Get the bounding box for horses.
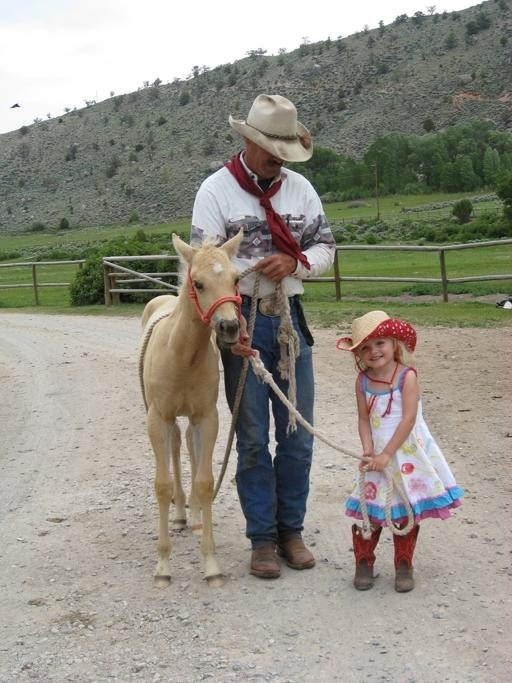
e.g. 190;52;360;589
138;226;246;591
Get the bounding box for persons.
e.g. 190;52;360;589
186;92;339;580
335;308;464;592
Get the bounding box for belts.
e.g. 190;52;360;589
249;295;296;318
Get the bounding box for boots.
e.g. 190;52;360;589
351;524;381;590
394;525;418;592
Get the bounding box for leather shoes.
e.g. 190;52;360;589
250;544;280;578
277;537;314;570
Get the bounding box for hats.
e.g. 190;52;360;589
337;311;418;353
228;94;314;163
